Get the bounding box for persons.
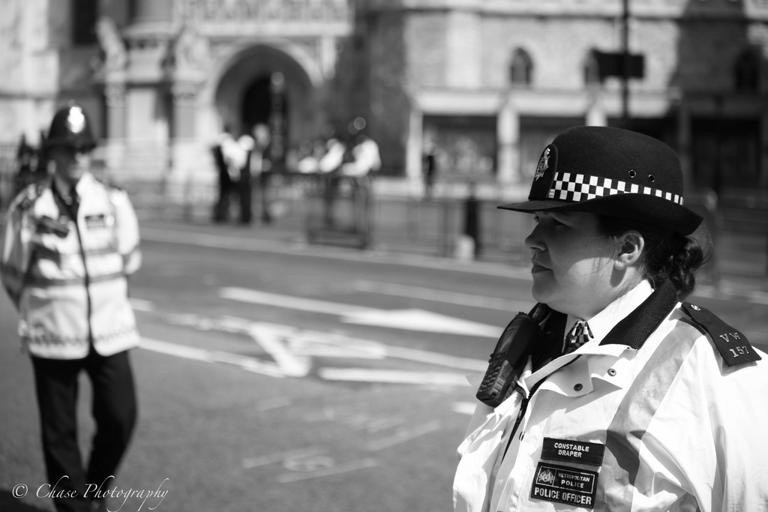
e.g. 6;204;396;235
13;132;51;195
210;121;381;249
462;181;482;261
421;143;440;198
451;126;768;512
0;103;143;512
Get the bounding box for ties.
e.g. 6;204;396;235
564;321;593;354
69;185;79;221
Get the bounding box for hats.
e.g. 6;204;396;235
497;127;704;238
45;106;96;150
